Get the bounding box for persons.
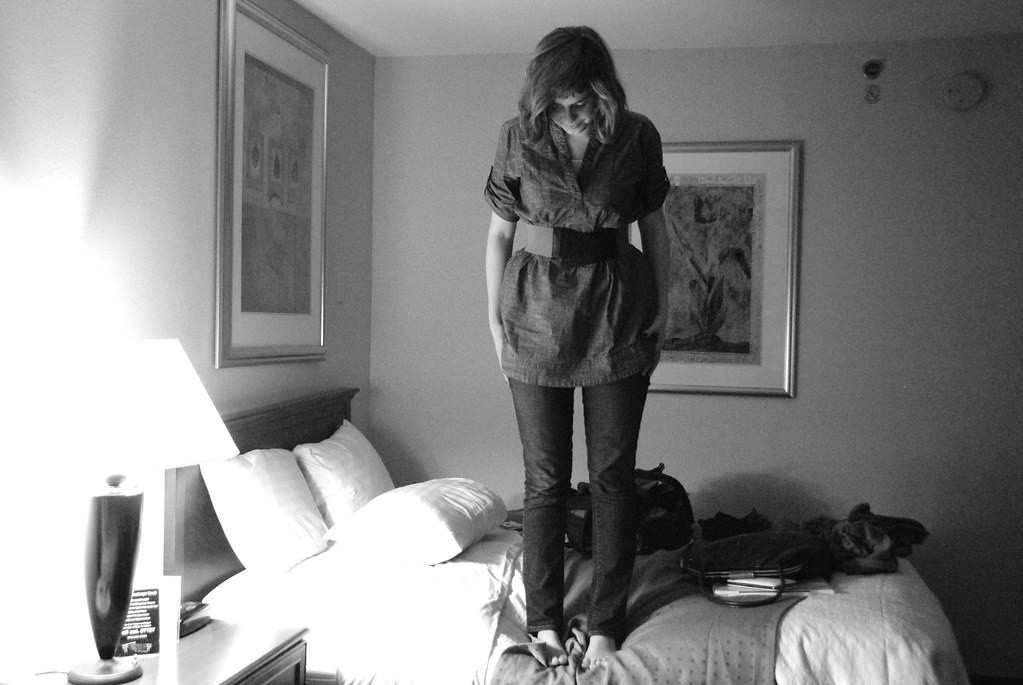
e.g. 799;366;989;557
486;26;671;670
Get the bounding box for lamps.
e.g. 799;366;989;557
57;337;239;685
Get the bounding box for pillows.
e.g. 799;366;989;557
322;477;508;567
292;418;395;530
200;448;330;577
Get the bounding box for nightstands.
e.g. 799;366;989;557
0;617;310;685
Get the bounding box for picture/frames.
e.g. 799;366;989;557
627;139;806;399
213;0;332;369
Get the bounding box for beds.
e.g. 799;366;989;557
163;387;969;685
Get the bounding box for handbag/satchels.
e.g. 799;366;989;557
685;530;832;607
565;465;694;558
